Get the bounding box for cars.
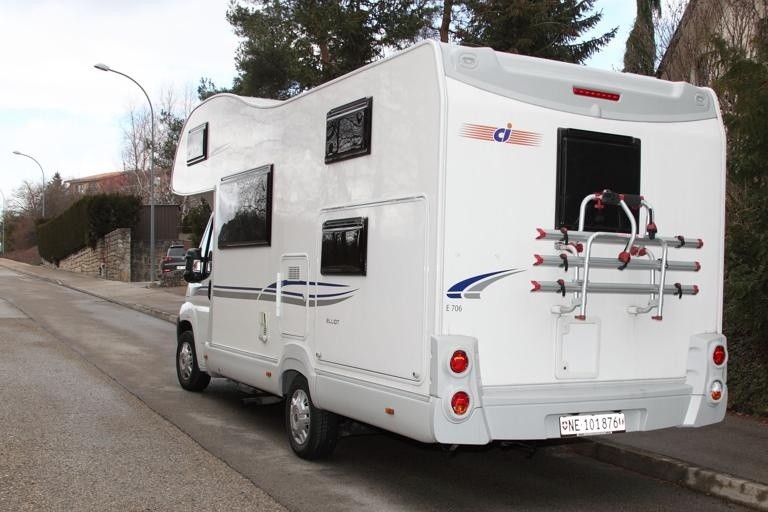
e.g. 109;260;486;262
160;245;187;279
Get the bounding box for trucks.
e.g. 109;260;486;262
170;38;728;461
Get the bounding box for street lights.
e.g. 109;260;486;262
94;62;156;282
13;151;45;216
